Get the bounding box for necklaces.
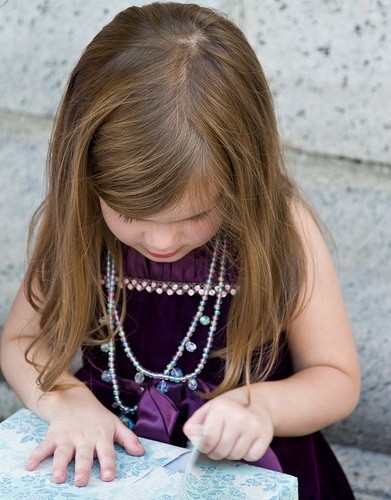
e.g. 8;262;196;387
98;231;229;429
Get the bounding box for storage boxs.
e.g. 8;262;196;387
0;407;298;500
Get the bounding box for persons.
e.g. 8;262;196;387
1;0;363;500
